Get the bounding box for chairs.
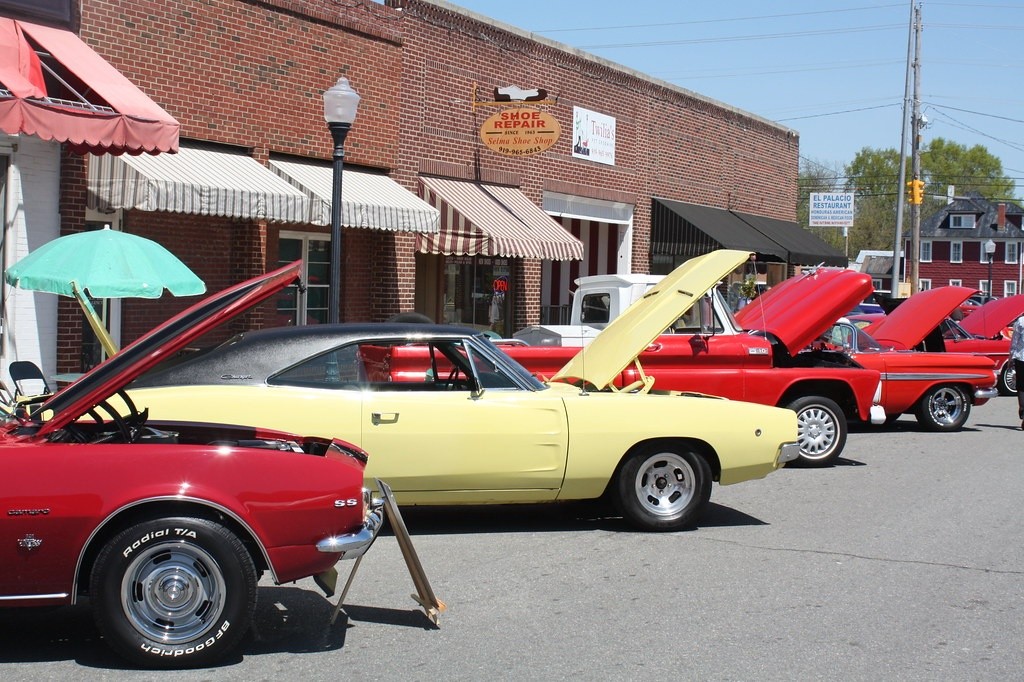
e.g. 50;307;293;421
8;361;55;406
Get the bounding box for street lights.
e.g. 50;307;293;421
320;75;361;325
985;238;996;300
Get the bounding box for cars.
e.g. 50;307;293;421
792;285;999;432
511;272;744;350
358;265;888;470
14;249;803;532
0;257;386;669
842;289;1024;398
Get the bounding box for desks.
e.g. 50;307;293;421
50;373;86;382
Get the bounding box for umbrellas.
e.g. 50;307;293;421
4;224;207;364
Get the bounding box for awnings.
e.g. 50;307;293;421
651;198;848;267
0;9;181;157
414;175;585;262
85;140;440;235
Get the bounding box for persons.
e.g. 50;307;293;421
736;285;772;313
1008;314;1024;430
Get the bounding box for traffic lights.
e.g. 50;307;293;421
906;180;925;205
